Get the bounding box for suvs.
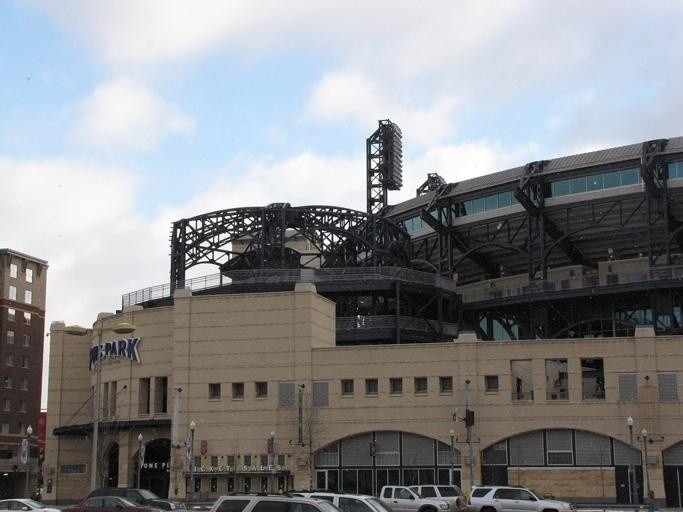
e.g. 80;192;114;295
293;491;393;511
86;487;175;510
465;485;576;512
210;495;341;511
407;484;466;511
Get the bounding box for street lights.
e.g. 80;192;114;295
269;430;276;493
626;415;639;511
23;424;32;497
61;322;135;491
189;420;195;508
641;427;652;492
137;433;143;488
447;430;455;484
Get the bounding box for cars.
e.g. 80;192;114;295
62;495;165;512
0;498;62;512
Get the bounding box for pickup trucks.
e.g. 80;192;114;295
379;484;451;512
